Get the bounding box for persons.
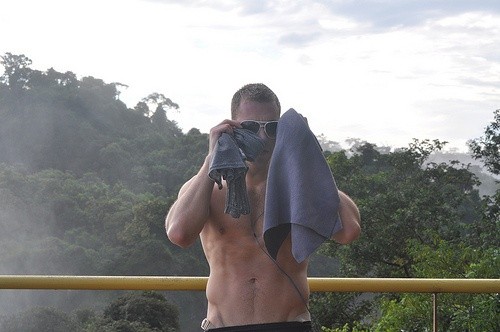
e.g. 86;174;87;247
165;84;361;332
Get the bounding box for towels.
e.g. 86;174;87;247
263;108;344;265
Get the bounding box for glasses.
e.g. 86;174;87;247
240;120;278;138
